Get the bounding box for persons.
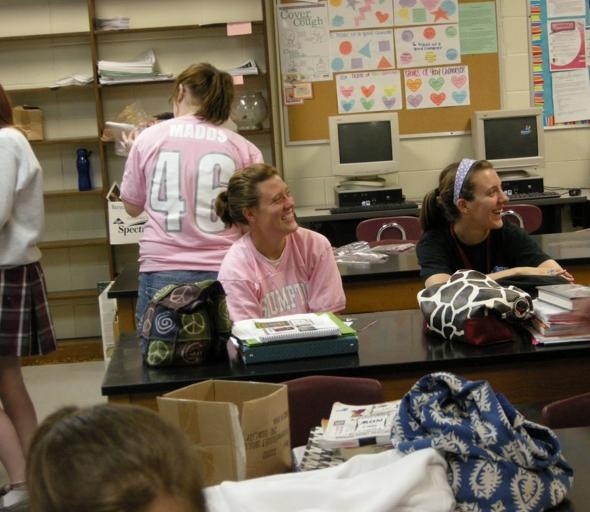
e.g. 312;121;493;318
416;154;570;285
209;164;346;322
0;81;63;507
116;60;267;333
20;404;213;512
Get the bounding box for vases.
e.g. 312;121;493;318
228;89;269;134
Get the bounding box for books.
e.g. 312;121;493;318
95;48;156;83
526;279;588;342
93;15;130;31
294;400;419;474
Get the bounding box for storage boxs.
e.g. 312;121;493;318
10;103;46;144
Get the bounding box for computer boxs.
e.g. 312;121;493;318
497;172;544;194
334;185;402;208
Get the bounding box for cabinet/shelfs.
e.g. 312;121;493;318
1;1;289;349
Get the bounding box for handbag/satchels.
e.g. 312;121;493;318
416;268;534;346
140;279;232;369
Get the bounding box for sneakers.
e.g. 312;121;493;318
1;491;30;511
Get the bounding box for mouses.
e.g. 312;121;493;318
565;186;582;196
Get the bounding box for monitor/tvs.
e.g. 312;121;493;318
328;110;401;186
471;106;545;177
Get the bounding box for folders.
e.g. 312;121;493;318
239;334;360;366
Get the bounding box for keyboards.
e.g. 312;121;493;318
506;190;561;202
330;200;419;214
